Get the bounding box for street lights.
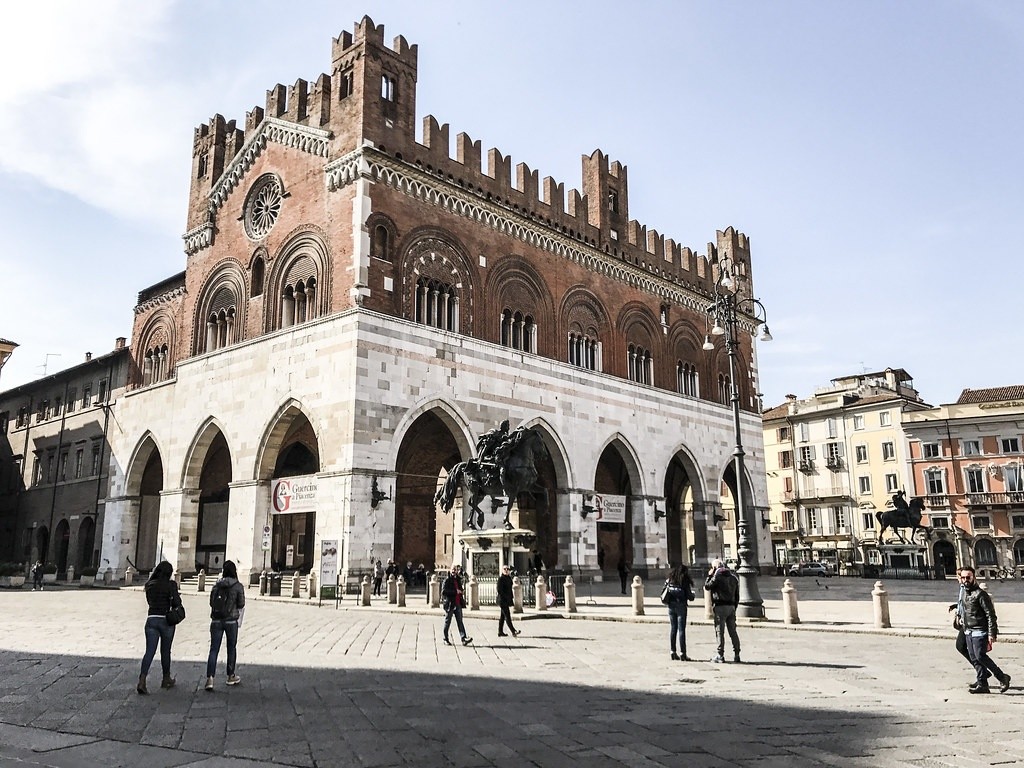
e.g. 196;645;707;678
703;251;773;619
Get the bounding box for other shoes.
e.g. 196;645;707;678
986;673;992;678
734;656;740;663
969;683;977;688
1000;674;1011;692
31;588;37;590
513;630;521;637
712;656;725;663
969;684;990;694
444;640;451;645
40;586;44;591
498;632;508;637
462;637;473;646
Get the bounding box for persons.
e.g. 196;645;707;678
403;562;413;591
704;559;741;664
496;565;521;637
949;566;1011;694
895;490;913;527
204;560;245;689
477;420;510;478
373;560;384;598
385;558;400;598
31;560;44;591
416;563;427;587
442;564;473;646
661;564;696;661
137;561;183;695
618;557;631;594
533;549;543;575
598;547;605;570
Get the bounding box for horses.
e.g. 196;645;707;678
876;497;932;545
434;429;550;530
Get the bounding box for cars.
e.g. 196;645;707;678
789;562;835;577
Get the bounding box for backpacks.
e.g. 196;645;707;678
210;581;239;620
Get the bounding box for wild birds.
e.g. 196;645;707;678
815;580;829;589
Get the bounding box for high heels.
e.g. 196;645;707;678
671;652;680;660
680;653;691;661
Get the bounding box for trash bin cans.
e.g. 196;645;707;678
935;565;946;580
267;573;281;596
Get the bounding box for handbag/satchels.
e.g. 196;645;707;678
165;604;185;626
660;577;672;605
624;560;631;574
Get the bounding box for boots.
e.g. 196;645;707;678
162;671;175;688
137;673;148;695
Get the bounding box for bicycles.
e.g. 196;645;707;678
998;566;1022;579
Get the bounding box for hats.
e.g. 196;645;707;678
387;558;395;564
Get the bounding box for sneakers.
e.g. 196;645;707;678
226;673;241;685
205;675;214;690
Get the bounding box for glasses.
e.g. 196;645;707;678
504;568;509;570
961;576;973;580
